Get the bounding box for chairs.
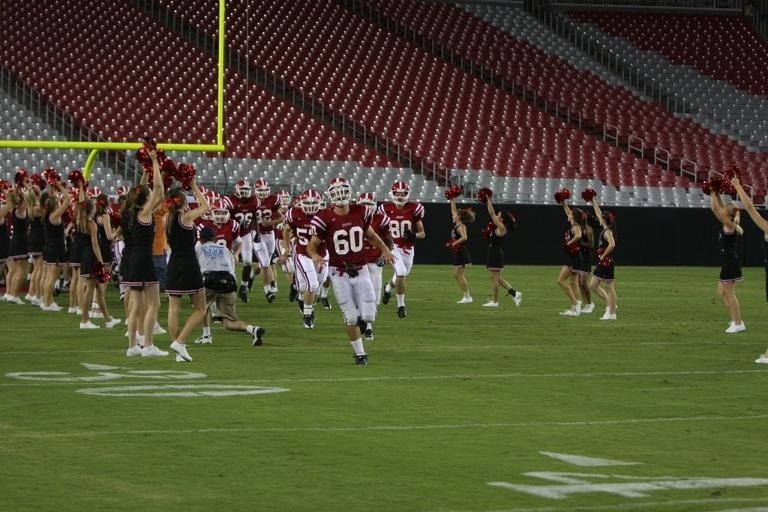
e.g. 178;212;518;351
0;0;767;209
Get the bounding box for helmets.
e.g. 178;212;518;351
234;177;271;198
118;185;131;195
197;189;232;223
389;181;411;205
278;178;378;216
87;187;100;198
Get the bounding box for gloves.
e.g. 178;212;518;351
403;228;416;240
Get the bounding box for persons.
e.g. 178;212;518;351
0;152;425;368
481;193;524;308
447;199;476;305
730;169;768;364
706;188;745;333
556;195;621;321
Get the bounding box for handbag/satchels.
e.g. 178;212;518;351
205;270;238;292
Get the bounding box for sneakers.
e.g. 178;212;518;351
559;300;618;320
725;320;746;332
363;328;373;338
289;280;331;328
251;326;266;346
513;291;522;307
3;293;63;311
457;296;473;304
382;282;391;304
482;302;499;307
397;305;406;318
353;354;370;365
122;316;214;363
239;274;277;304
68;302;121;329
